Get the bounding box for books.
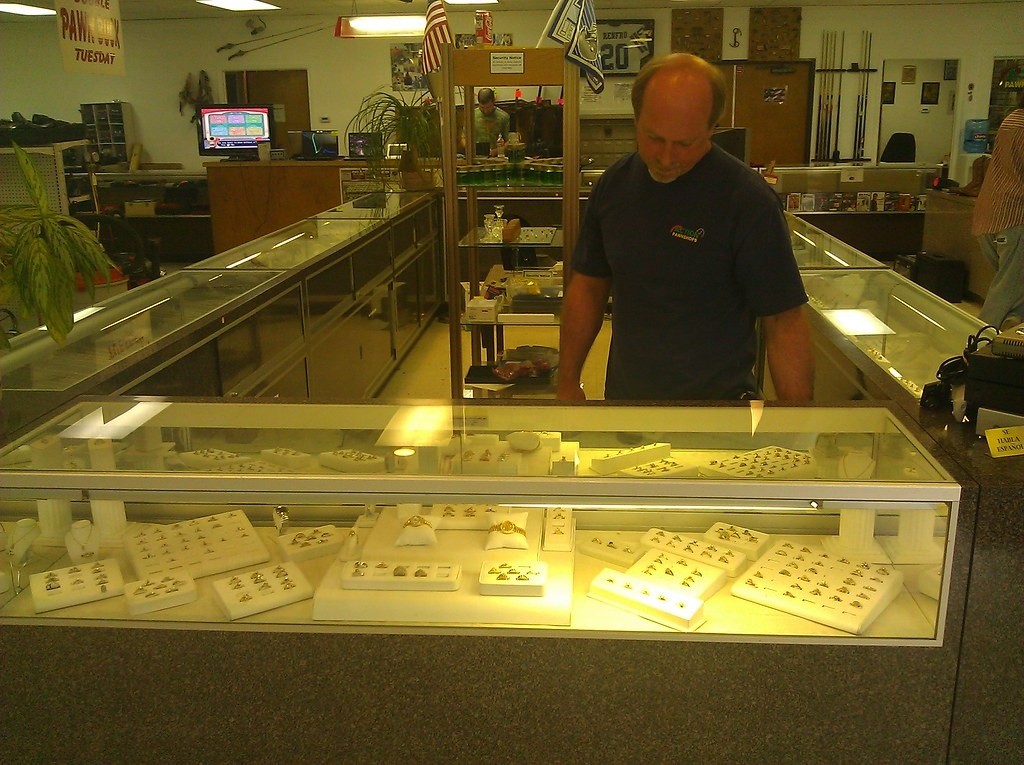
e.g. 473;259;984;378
778;192;927;211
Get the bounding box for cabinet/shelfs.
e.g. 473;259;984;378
80;102;132;162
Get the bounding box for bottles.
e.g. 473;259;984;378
483;214;495;235
497;133;505;158
963;118;989;153
516;89;521;100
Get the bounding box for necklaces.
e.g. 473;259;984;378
70;525;93;551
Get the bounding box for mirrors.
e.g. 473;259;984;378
450;84;568;400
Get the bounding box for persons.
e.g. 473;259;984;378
971;103;1024;331
870;193;879;211
461;87;510;155
554;52;816;404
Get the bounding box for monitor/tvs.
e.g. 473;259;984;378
196;102;276;162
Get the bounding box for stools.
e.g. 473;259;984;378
73;265;131;304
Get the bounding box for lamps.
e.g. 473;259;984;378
340;0;427;40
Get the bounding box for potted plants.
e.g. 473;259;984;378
345;77;448;191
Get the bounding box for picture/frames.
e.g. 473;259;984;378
943;60;959;80
881;81;896;104
920;81;940;105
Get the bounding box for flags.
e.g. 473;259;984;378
547;0;604;94
421;0;452;75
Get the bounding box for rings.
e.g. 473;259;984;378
606;576;685;608
291;529;330;547
45;561;109;593
553;506;566;534
442;505;494;517
489;563;535;581
643;553;703;587
872;348;921;393
133;575;184;597
229;564;296;603
133;511;250;559
591;536;633;554
174;430;815;477
351;560;428;577
652;525;888;608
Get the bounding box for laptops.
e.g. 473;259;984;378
287;130;339;160
343;132;383;160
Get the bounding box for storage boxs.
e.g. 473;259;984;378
465;297;497;322
124;201;156;217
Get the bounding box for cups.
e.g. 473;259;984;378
509;132;521;144
491;218;508;239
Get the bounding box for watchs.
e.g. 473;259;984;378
488;520;526;537
274;506;290;535
403;515;433;530
